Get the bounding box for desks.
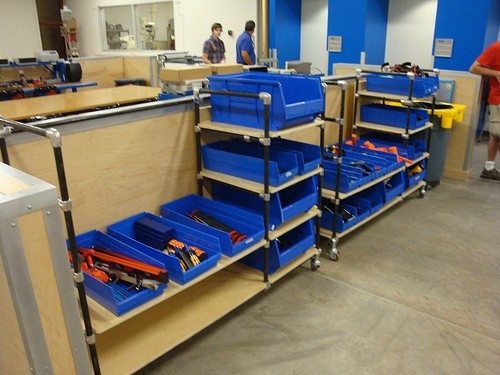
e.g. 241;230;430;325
44;80;96;91
0;84;162;121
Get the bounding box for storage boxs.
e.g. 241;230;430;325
65;64;438;316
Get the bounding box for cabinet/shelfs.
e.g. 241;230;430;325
314;68;441;261
0;82;327;375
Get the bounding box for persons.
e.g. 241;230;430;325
469;39;500;180
236;19;257;65
202;22;227;65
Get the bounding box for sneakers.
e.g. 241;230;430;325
480;168;500;180
